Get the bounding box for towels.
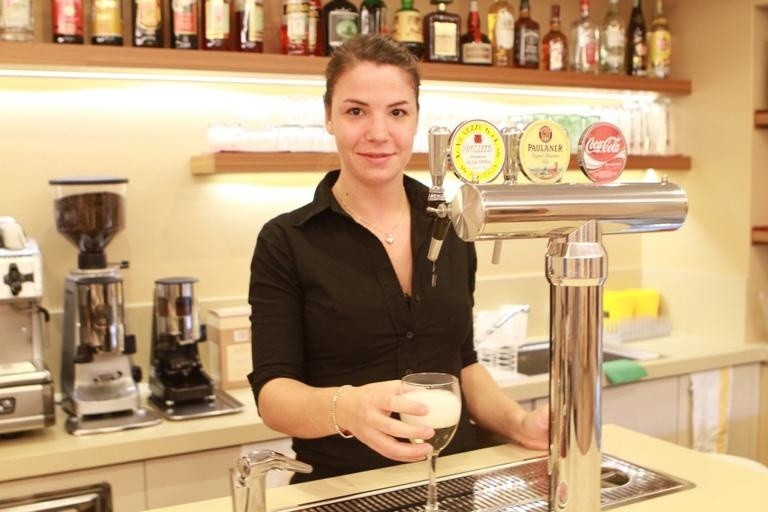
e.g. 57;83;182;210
690;366;731;456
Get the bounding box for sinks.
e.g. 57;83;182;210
481;338;661;376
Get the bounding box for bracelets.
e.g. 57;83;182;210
330;384;354;440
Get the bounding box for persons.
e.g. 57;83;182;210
246;33;551;484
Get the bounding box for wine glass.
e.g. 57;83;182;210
398;372;463;512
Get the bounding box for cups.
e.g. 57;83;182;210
473;310;501;353
630;285;659;321
506;110;599;155
497;302;528;347
603;289;635;332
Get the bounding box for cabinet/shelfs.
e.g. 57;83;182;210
0;35;696;176
530;359;759;465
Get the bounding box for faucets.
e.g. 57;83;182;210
473;304;533;349
425;126;452;263
228;447;314;512
491;125;522;267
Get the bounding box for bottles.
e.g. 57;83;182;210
1;0;675;79
613;90;667;155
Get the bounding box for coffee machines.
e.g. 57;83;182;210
46;175;147;420
146;275;215;409
0;216;58;438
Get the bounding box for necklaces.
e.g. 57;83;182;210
334;184;406;245
